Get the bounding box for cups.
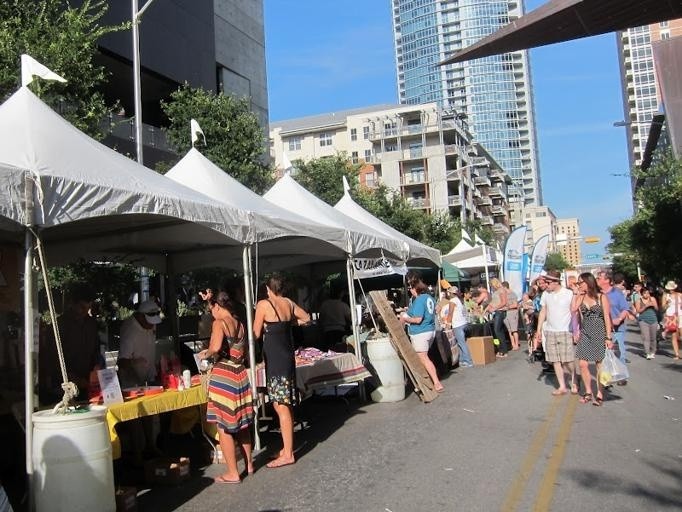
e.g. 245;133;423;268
183;369;191;389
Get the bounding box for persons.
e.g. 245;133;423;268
116;298;163;460
192;286;257;483
252;274;311;470
46;284;110;401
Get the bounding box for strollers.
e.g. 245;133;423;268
524;311;547;363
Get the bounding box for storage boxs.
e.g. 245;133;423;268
208;438;242;464
143;455;190;483
115;486;138;512
466;335;499;365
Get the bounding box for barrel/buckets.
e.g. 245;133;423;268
30;406;118;512
366;337;406;403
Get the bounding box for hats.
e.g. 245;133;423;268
543;271;562;282
446;286;459;294
138;301;162;325
665;280;678;290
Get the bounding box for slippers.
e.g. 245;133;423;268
594;393;604;406
265;456;296;468
552;387;567;395
571;385;580;394
580;392;593;403
214;474;241;484
273;449;293;457
245;465;256;475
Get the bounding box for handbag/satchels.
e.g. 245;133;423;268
664;313;679;332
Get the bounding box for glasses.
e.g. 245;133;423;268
145;310;160;316
575;280;585;285
543;279;553;284
407;287;412;292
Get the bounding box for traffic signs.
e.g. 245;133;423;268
584;253;606;259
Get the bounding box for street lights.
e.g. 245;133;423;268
429;159;497;221
546;236;602;250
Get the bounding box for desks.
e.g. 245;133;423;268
103;374;224;468
252;350;372;446
